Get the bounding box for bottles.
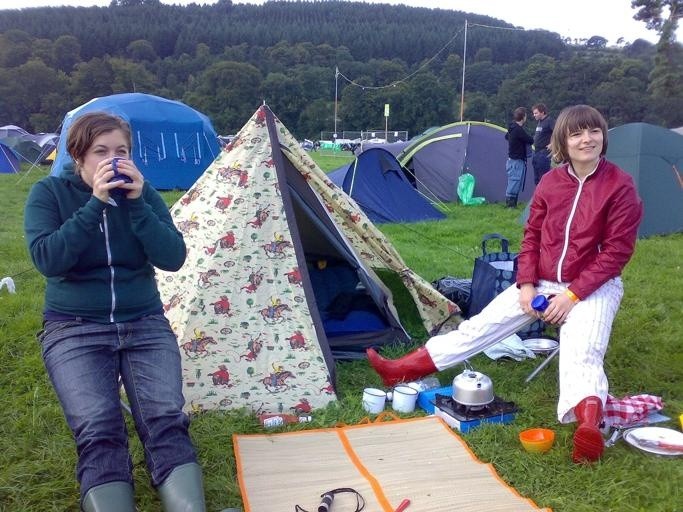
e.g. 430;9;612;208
387;377;442;402
530;294;565;329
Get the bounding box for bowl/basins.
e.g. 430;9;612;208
519;428;555;456
521;337;560;355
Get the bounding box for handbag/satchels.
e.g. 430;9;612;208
430;232;546;342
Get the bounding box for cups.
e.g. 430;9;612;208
105;156;134;197
359;386;419;415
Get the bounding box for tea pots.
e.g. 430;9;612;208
453;360;495;406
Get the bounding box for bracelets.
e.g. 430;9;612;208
564;289;582;304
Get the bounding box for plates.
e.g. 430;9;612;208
624;427;683;458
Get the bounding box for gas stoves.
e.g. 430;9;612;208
430;391;517;421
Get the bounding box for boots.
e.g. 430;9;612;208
505;197;517;208
366;345;439;388
573;395;604;464
82;462;206;512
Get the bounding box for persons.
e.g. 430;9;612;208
23;111;208;511
503;105;535;211
365;105;646;462
529;103;555;188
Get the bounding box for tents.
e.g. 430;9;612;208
603;121;683;240
1;124;61;176
116;100;469;422
217;132;404;152
49;92;224;191
326;147;448;224
397;119;553;205
